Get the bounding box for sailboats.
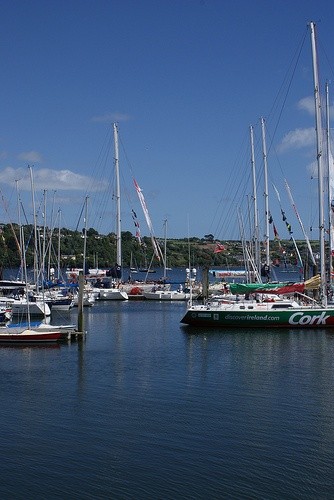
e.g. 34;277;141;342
0;21;334;344
